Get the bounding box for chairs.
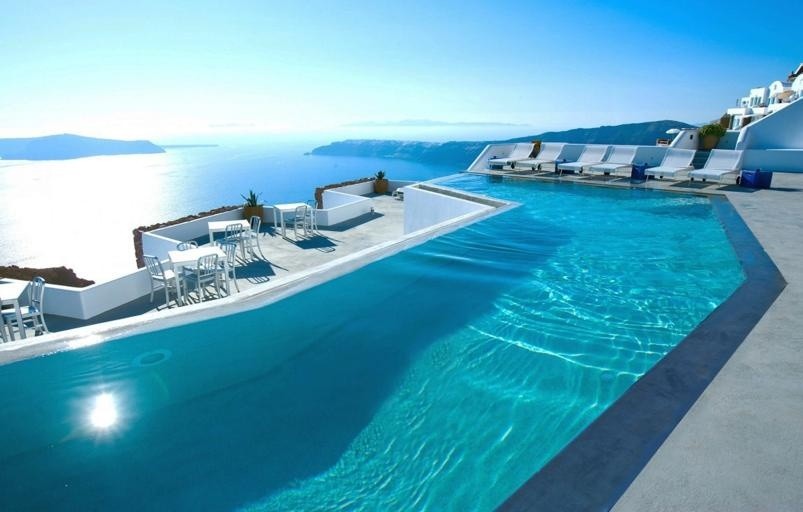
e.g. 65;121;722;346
141;215;263;308
488;143;744;190
283;199;318;240
2;276;49;342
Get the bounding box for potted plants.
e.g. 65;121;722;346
373;171;388;195
699;123;725;151
239;189;263;221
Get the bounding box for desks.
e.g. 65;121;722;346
0;281;31;343
272;203;313;238
